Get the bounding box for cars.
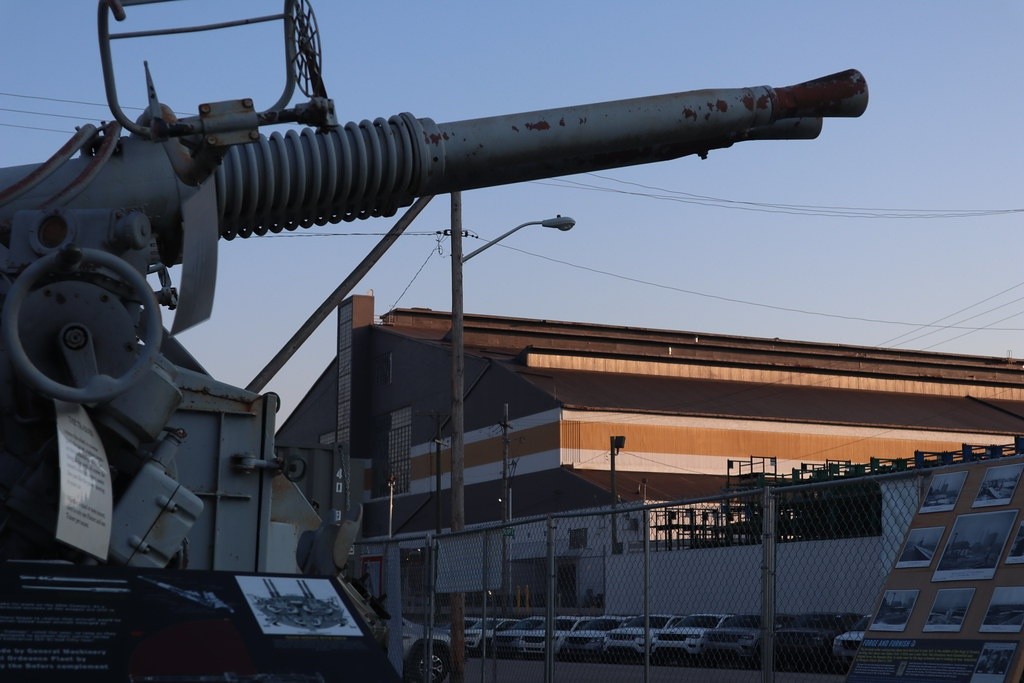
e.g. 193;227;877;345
463;619;520;658
401;617;468;683
443;618;483;635
773;612;864;673
833;614;872;674
652;614;736;667
603;615;689;664
491;617;546;659
700;614;789;669
561;616;639;662
518;617;601;660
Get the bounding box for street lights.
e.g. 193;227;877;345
611;436;626;554
448;214;576;683
